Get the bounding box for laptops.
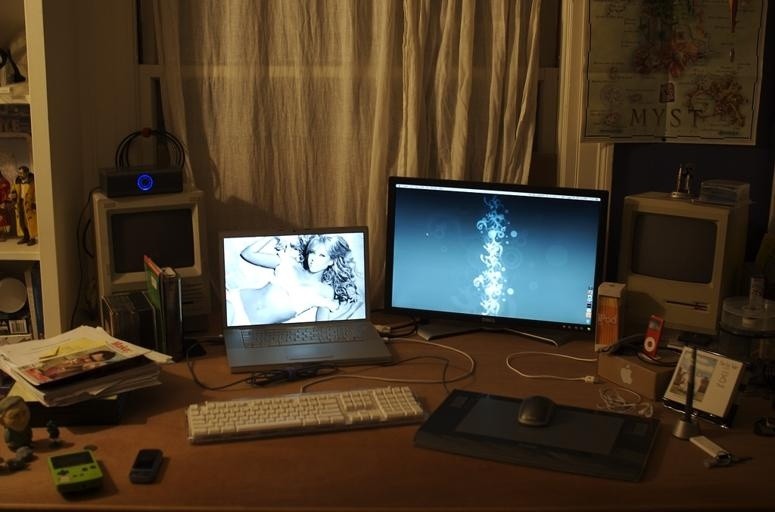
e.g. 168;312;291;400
217;225;393;375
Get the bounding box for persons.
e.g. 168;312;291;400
0;171;11;240
2;165;37;247
226;232;365;328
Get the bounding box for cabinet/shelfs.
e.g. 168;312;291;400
0;0;139;345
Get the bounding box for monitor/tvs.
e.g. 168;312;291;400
90;188;211;317
617;191;749;354
384;176;610;349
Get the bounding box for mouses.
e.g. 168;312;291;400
517;394;556;427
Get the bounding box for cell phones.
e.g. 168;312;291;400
128;449;164;483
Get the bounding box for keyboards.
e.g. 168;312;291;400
184;385;431;446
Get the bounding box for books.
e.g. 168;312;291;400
0;254;187;425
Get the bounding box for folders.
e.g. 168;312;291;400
143;254;167;355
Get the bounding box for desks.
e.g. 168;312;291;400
0;330;775;511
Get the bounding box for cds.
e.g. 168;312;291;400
721;295;775;332
